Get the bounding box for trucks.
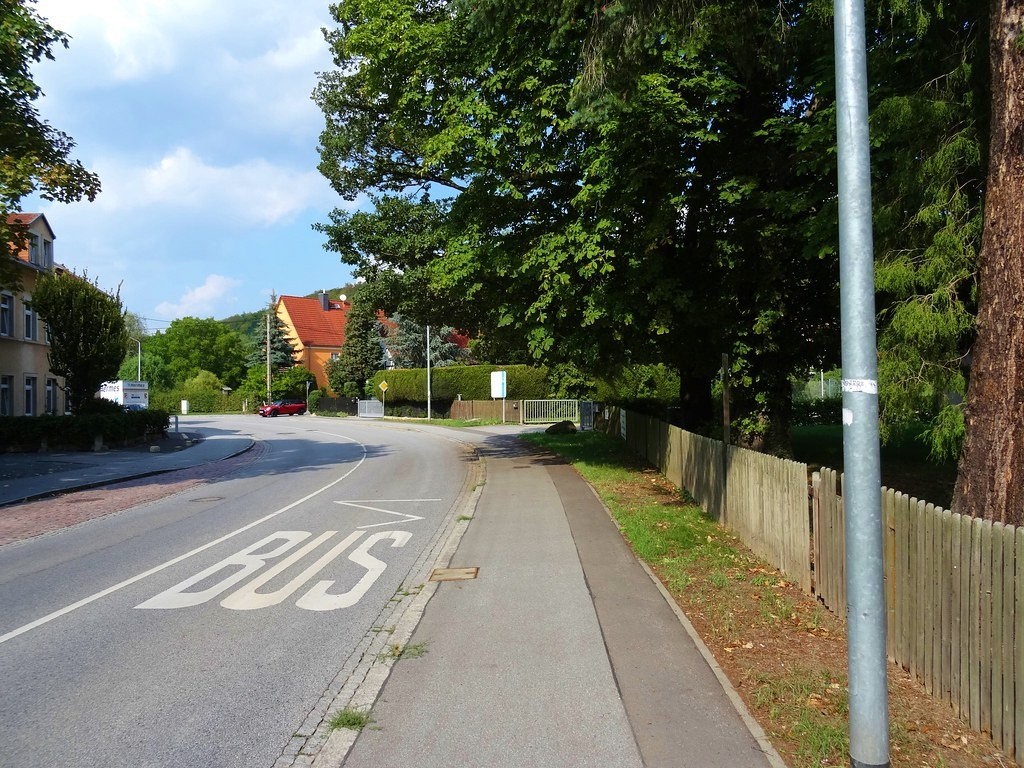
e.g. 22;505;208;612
99;380;149;412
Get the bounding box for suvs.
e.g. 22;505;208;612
259;399;306;417
